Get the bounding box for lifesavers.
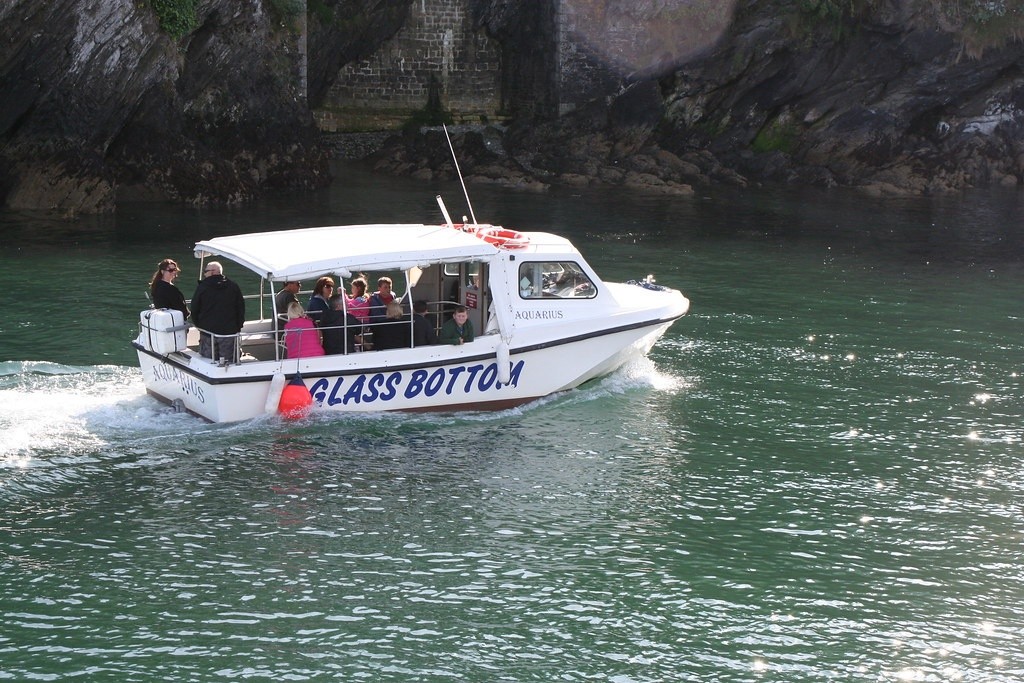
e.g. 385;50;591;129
476;227;530;248
441;223;493;233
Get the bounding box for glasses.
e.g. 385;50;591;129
291;281;301;287
322;283;334;288
165;268;177;272
205;269;213;273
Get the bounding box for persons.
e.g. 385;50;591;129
189;262;246;362
152;259;190;333
438;307;474;345
271;275;438;357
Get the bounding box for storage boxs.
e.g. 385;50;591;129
140;307;186;353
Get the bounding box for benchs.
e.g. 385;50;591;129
240;319;274;346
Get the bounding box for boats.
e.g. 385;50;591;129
128;124;691;426
